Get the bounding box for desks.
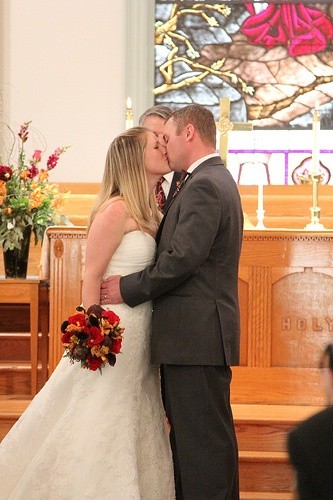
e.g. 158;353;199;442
0;279;49;399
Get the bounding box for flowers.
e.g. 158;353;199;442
61;304;126;374
0;121;72;250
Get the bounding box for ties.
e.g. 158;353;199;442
154;178;166;210
172;171;190;198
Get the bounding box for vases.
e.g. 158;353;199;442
3;226;32;280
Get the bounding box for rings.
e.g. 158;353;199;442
105;295;107;298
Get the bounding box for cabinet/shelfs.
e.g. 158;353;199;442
48;226;333;405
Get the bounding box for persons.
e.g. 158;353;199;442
100;106;244;500
0;126;175;500
285;343;333;500
139;106;174;213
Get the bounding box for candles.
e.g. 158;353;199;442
304;100;326;174
124;95;134;129
256;183;266;229
